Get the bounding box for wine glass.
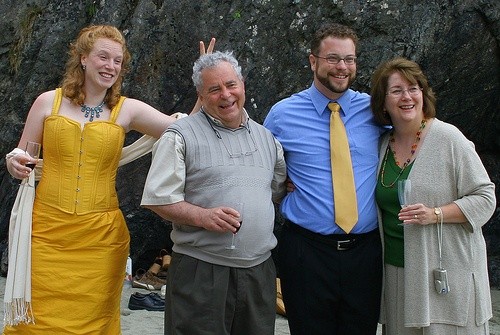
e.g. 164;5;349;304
397;179;414;227
18;141;41;188
225;201;244;250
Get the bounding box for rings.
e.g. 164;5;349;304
415;215;417;218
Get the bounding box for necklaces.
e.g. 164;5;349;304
381;119;426;187
80;101;105;121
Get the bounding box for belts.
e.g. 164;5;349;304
286;222;358;250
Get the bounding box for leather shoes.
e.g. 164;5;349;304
128;291;167;311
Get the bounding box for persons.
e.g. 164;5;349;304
4;23;216;334
262;22;393;335
139;52;287;335
285;57;497;335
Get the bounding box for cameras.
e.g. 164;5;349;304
434;268;450;295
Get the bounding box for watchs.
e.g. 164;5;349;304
435;208;441;224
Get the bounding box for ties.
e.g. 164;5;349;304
328;99;358;234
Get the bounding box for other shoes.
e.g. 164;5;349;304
132;268;167;291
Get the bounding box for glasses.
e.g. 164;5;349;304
220;132;258;159
382;86;423;98
311;52;357;69
